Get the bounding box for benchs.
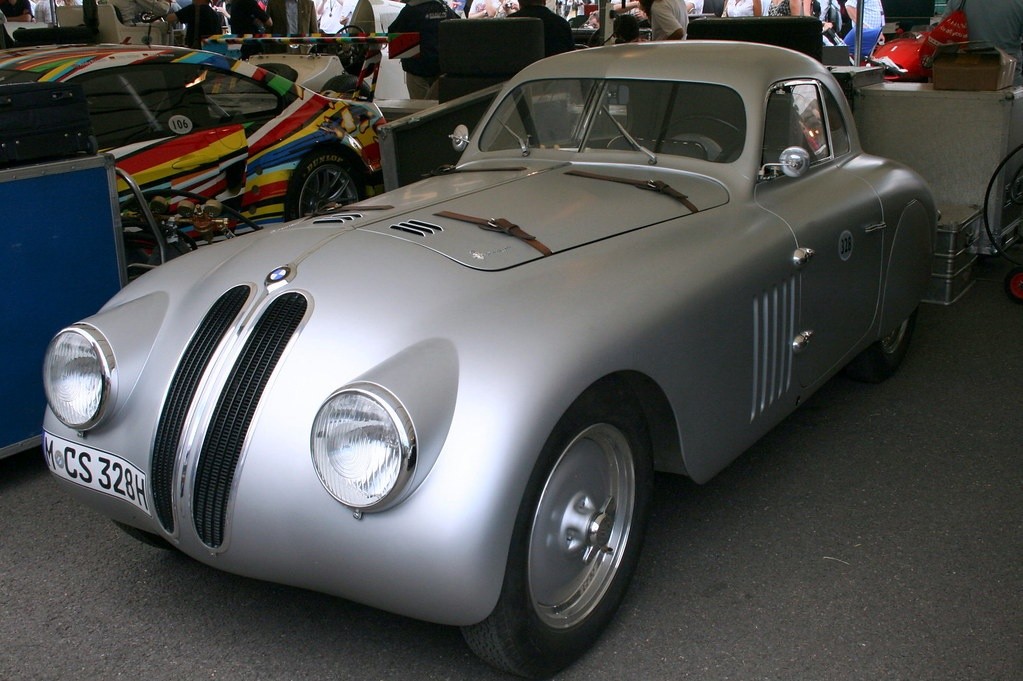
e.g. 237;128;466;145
55;4;162;45
628;85;798;169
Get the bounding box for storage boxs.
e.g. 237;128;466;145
920;205;983;307
932;40;1017;90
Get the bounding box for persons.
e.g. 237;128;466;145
0;0;1023;100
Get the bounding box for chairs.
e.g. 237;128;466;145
439;16;545;102
686;17;824;72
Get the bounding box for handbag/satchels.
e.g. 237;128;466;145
919;10;969;69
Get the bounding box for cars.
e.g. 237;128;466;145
39;38;942;681
0;45;389;288
244;0;410;100
